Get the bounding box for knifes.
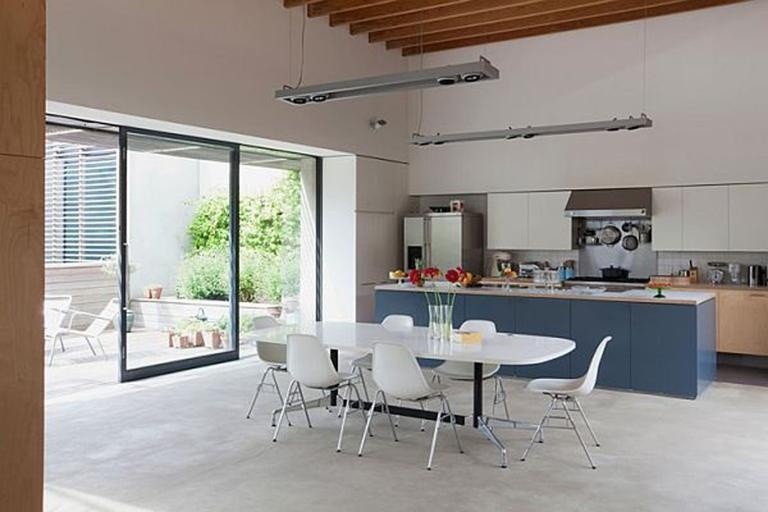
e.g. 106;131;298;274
688;260;693;270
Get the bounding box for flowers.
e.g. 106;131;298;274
392;265;472;303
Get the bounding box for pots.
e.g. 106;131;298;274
598;264;631;279
576;218;652;251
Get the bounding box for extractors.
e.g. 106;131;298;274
561;188;652;218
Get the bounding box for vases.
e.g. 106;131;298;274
428;306;453;343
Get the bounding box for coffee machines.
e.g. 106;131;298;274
489;250;517;277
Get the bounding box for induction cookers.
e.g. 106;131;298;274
565;273;650;285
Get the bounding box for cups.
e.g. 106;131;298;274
678;269;688;277
747;264;761;286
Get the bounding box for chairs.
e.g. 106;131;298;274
421;318;517;432
338;313;414;427
522;336;614;469
357;341;465;470
245;314;325;429
44;294;72;351
270;333;374;452
44;298;123;368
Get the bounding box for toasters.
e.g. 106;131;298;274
518;260;549;278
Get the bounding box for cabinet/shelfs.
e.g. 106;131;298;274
319;156;420;324
653;275;768;358
651;182;767;253
484;192;582;251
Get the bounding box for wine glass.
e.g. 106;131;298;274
648;273;673;299
497;274;514;296
532;269;561;296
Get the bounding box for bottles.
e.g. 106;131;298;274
564;260;573;278
705;261;729;288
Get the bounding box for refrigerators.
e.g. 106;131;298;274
401;211;483;278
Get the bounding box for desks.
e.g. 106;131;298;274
236;320;578;429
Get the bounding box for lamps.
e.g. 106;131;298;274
271;0;500;107
410;0;654;147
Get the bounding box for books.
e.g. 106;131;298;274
451;329;483;346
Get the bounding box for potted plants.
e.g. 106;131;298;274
160;306;231;351
104;254;137;332
150;282;161;299
143;284;151;298
